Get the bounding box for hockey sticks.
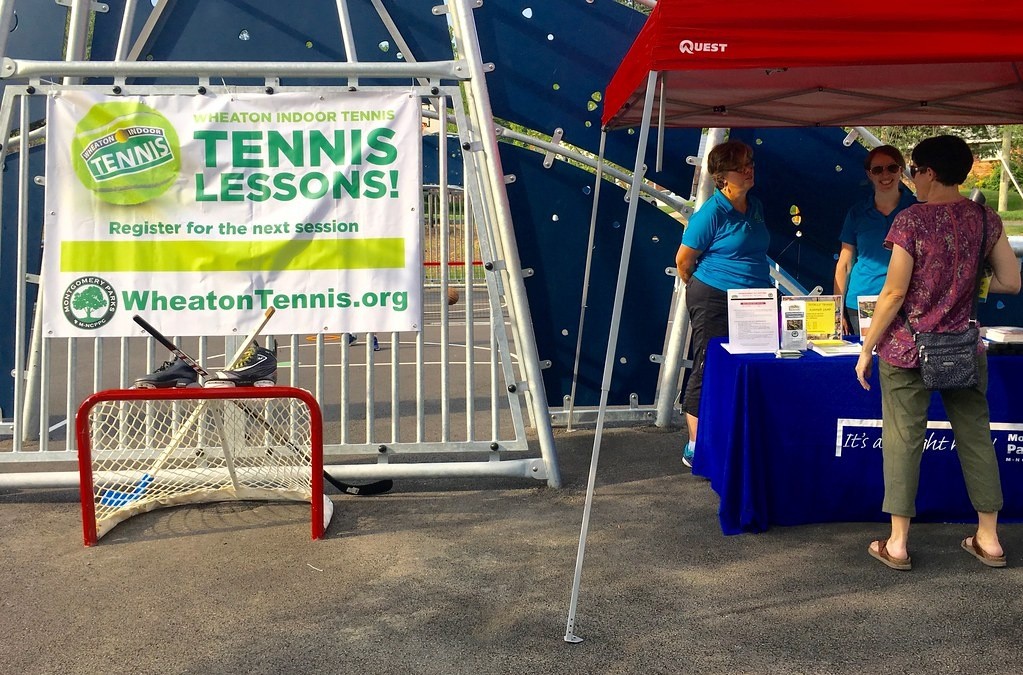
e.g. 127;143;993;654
132;314;393;495
99;305;276;509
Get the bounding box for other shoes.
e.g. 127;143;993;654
374;335;380;351
348;333;357;346
682;443;695;467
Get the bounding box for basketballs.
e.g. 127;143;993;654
448;286;460;305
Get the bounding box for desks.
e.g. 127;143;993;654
698;335;1023;536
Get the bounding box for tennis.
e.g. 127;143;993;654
70;99;182;207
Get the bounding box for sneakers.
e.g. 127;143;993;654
209;341;277;387
135;354;197;390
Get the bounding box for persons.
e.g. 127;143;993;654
854;134;1022;567
674;140;772;468
833;144;922;336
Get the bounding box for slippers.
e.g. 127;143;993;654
961;529;1006;567
868;539;912;570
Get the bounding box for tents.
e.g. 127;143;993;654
563;1;1020;646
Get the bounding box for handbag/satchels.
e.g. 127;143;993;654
913;328;979;390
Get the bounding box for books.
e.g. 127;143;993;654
978;265;994;303
980;326;1023;347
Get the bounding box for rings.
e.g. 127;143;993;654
857;378;860;381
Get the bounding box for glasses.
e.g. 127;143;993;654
910;165;938;179
868;164;902;176
721;160;755;174
